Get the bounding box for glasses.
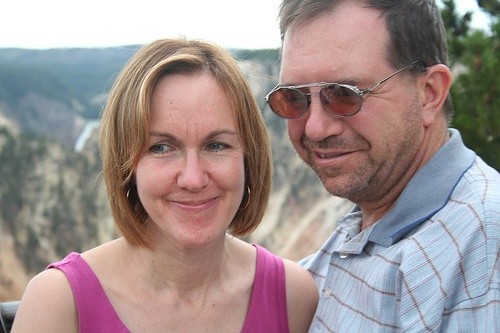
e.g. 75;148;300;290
264;60;420;118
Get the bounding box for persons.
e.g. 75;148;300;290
9;38;318;333
265;0;499;332
